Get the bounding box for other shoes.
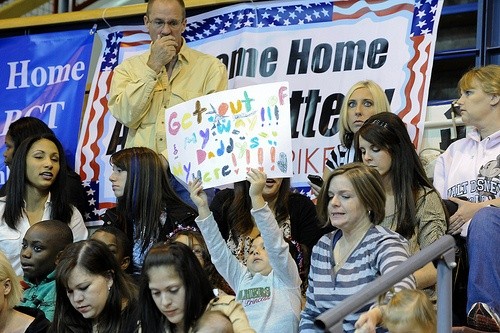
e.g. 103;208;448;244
467;301;500;331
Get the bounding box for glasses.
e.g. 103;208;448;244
192;247;206;256
148;19;182;28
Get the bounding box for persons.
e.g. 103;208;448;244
168;229;258;333
298;161;418;333
208;177;324;297
0;249;55;333
0;132;88;278
307;79;391;197
383;290;437;333
0;116;92;222
432;64;500;333
101;146;200;277
16;220;74;323
353;111;449;314
188;167;302;333
136;241;234;333
47;238;142;333
107;0;228;162
